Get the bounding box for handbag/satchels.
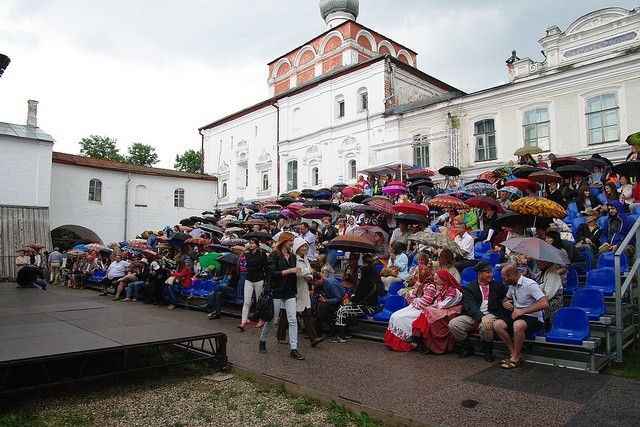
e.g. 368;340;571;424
258;265;276;321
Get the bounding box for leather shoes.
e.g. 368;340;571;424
54;284;58;285
208;315;220;319
278;340;288;344
238;325;245;331
49;281;52;284
421;348;429;355
311;338;323;346
254;322;265;328
259;340;267;354
290;349;305;360
199;303;212;311
404;336;418;343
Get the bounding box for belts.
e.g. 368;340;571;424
51;262;60;263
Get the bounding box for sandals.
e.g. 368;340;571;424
501;360;520;369
501;355;522;364
99;291;107;296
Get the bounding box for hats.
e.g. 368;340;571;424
474;261;491;272
580;210;599;216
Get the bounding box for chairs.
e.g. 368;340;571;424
631;205;639;213
596;215;610;230
491;266;503;281
596;193;607;204
589;188;599;197
182;279;201;294
525;310;545;340
430;224;440;232
362;297;387;320
227;277;244;303
561;209;575;224
406;251;429;271
572;251;592;274
596;251;630;274
571;216;586;233
562;268;580;295
567;288;607;321
372;294;406;322
336;249;346;258
627;213;639;221
566;201;580;216
468;230;485;238
544;306;591;345
584;267;615;297
88;270;105;280
473;241;492;259
615;180;621;188
481;251;500;270
566;227;580;244
375;264;384;273
459;266;479;287
203;281;219;297
334;276;343;282
377;282;406;303
192;279;212;297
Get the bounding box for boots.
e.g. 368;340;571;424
482;341;494;362
457;336;473;358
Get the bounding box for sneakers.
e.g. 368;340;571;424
121;298;131;302
132;298;137;302
